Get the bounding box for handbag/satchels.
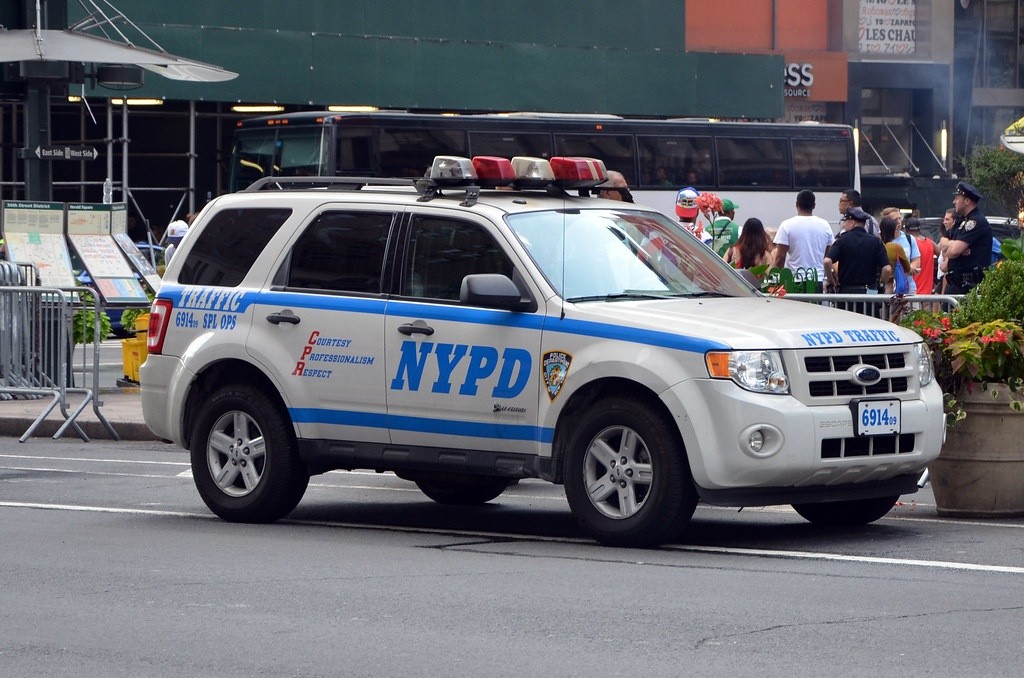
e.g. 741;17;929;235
894;262;910;295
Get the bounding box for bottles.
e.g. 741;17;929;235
103;178;112;204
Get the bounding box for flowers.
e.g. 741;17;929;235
899;310;1024;428
685;191;788;299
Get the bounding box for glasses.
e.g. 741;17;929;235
839;198;850;203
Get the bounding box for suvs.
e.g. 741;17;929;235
140;158;946;546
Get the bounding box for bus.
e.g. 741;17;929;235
230;109;860;257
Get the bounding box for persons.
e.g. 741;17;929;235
596;170;631;201
164;220;188;270
686;171;697;183
675;181;1001;319
653;166;672;186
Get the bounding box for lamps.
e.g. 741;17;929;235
940;119;948;166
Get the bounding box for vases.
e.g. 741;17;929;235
928;383;1024;520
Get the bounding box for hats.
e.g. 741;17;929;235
717;199;740;212
903;218;921;232
675;186;700;218
953;182;984;204
167;220;189;238
840;207;871;223
